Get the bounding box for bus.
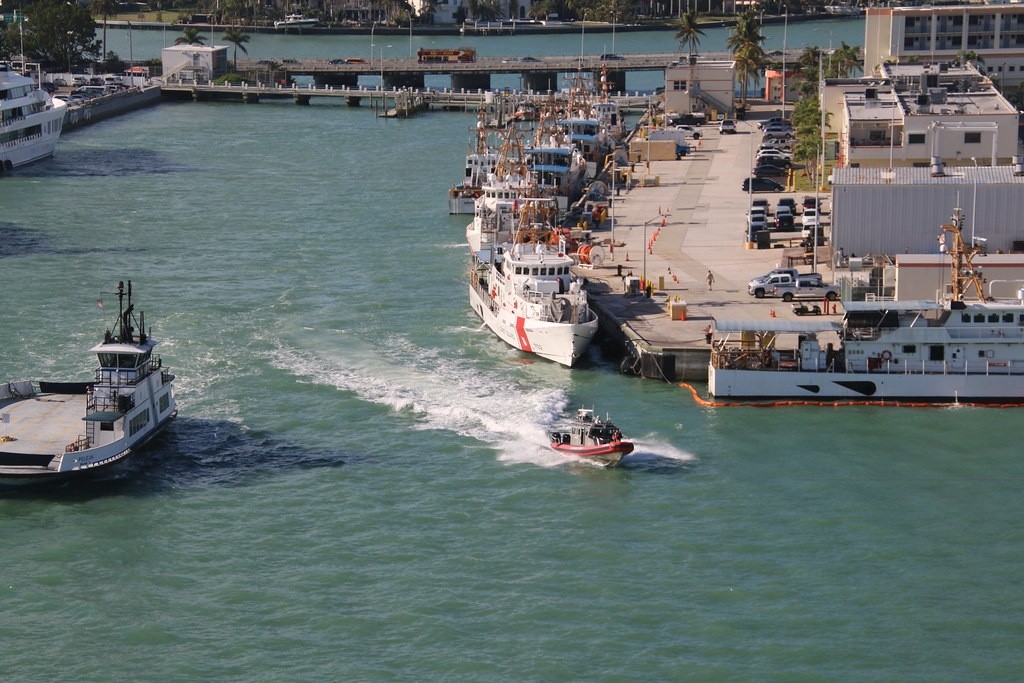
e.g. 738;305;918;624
417;47;477;63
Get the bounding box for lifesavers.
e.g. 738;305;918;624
881;348;892;361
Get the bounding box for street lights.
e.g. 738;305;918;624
582;13;587;62
67;31;73;72
610;10;615;54
371;21;376;60
406;9;411;57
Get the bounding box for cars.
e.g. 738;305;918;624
756;156;794;169
33;83;123;107
328;59;344;64
741;178;785;193
72;77;87;87
345;58;370;64
282;59;297;64
756;150;794;161
105;76;123;86
801;222;820;239
774;214;796;232
752;165;785;178
744;196;798;243
520;57;542;62
53;78;67;87
801;198;823;214
801;209;821;225
755;117;793;154
256;60;270;64
600;54;624;61
89;78;105;86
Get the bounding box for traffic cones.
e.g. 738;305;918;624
698;141;700;146
772;309;776;317
769;310;772;315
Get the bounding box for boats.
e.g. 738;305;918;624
0;71;67;172
468;197;598;367
548;405;634;468
446;100;624;258
0;280;179;486
707;290;1023;399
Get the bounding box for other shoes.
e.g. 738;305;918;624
709;289;712;291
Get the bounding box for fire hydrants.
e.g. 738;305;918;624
831;304;837;313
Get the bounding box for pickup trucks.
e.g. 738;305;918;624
774;278;841;302
751;268;822;283
669;114;706;128
719;119;737;134
677;125;703;140
747;274;796;298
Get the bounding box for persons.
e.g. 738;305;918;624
702;324;712;344
612;428;623;441
705;270;715;290
